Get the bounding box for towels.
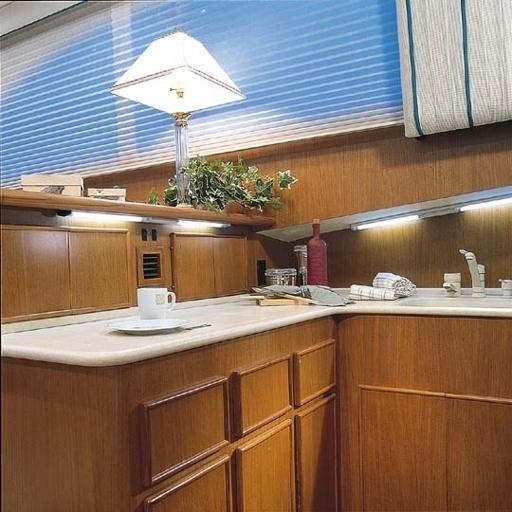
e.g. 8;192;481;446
348;284;396;302
257;284;355;306
371;273;416;297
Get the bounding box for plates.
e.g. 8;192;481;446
110;319;191;335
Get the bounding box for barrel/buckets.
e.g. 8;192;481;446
294;244;307;286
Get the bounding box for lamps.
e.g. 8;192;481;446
109;32;247;210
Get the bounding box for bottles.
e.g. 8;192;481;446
293;218;329;290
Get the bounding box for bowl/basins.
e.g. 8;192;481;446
263;268;296;288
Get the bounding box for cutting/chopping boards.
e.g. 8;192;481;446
255;297;310;307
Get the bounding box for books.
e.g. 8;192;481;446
256;298;309;306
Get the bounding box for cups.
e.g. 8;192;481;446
137;287;177;320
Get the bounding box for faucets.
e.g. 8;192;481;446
464;251;486;292
